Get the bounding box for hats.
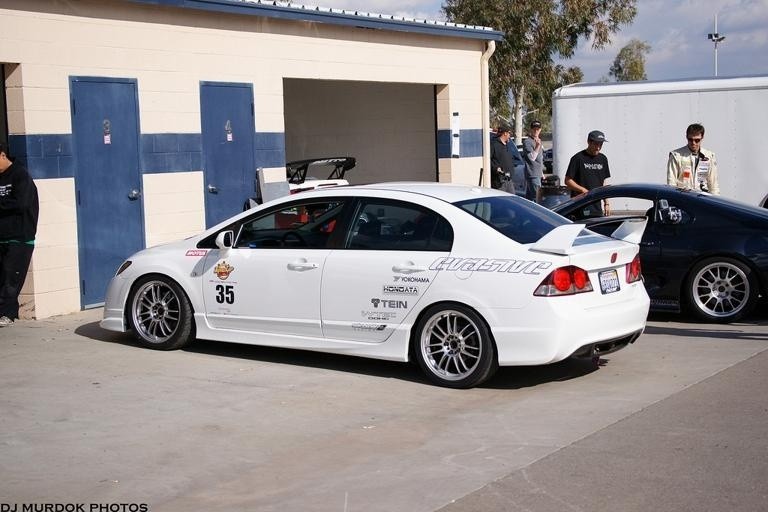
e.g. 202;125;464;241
588;130;608;142
492;126;511;138
531;121;542;129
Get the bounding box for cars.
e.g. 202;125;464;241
548;183;768;324
491;127;553;199
99;180;651;389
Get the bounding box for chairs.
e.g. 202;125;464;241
351;218;390;249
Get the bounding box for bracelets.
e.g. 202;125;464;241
603;203;608;206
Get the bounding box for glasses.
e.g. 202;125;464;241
687;137;702;143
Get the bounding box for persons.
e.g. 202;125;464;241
522;120;545;206
666;124;719;196
564;129;609;220
0;132;39;326
489;125;515;196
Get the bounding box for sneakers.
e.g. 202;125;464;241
0;316;14;327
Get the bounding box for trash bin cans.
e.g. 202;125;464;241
541;185;572;209
